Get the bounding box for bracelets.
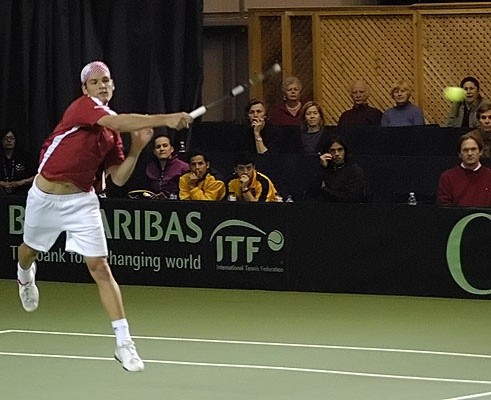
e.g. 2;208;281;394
241;188;249;193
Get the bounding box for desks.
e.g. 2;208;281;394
0;123;491;300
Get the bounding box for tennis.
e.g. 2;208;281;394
448;87;464;101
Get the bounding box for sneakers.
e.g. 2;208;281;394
17;261;39;312
113;342;145;372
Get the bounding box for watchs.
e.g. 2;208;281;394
255;137;262;142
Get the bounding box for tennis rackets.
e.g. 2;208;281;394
188;64;281;119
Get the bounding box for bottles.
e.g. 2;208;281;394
407;193;417;205
179;141;186;153
285;195;294;202
228;191;238;201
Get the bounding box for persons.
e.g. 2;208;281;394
179;152;226;200
378;84;425;128
143;134;192;198
437;134;491;207
291;100;348;158
0;128;35;194
227;155;279;202
235;99;273;157
16;62;194;372
274;77;304;126
309;135;366;202
338;85;382;128
447;77;491;128
464;104;491;155
